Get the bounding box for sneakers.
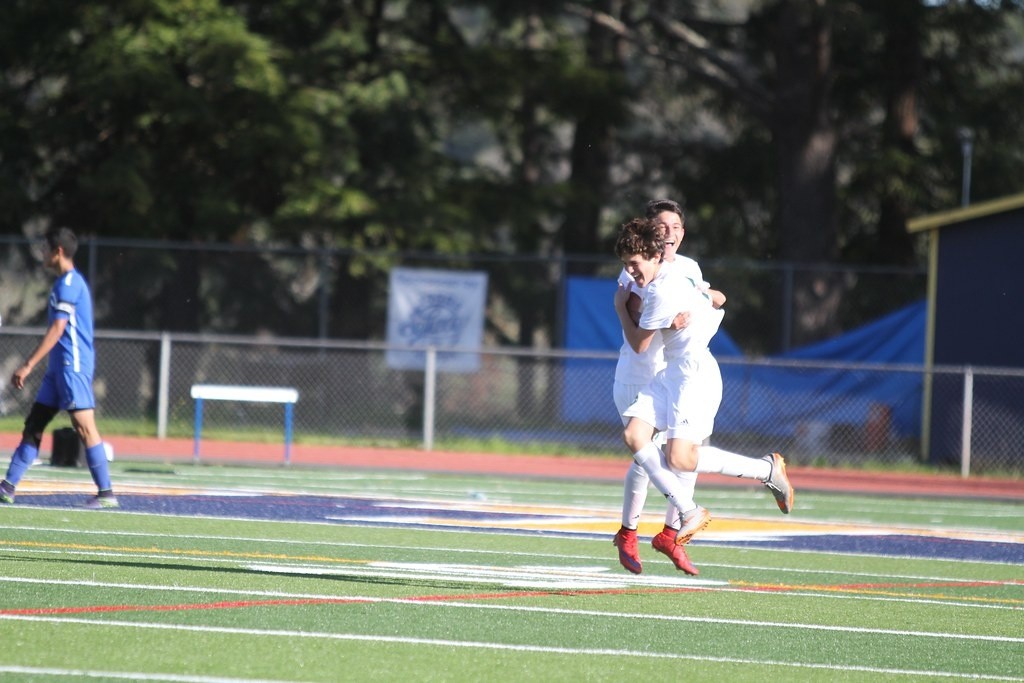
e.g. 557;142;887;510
82;489;118;509
760;452;794;514
651;525;700;576
612;526;642;574
674;504;712;545
0;480;16;503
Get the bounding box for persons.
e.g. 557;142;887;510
612;197;793;576
0;226;118;508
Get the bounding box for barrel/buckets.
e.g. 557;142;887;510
52;428;78;468
